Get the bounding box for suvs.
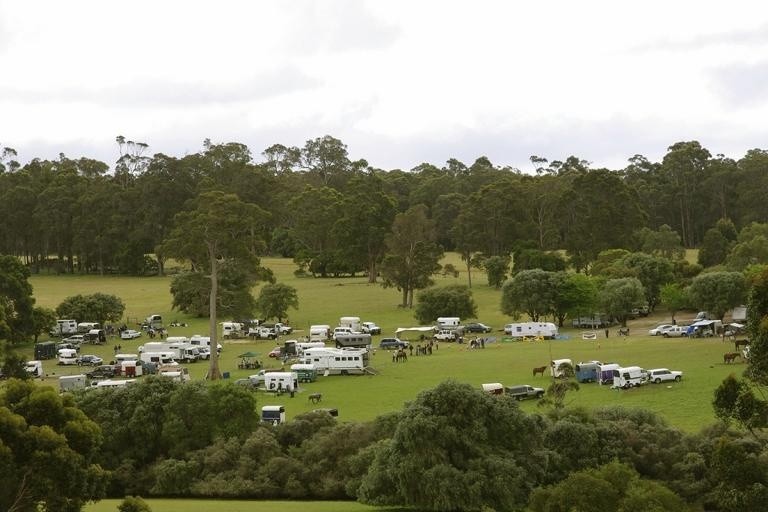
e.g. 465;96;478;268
646;368;683;384
463;322;493;334
504;324;512;335
379;337;410;350
649;325;672;336
433;330;457;342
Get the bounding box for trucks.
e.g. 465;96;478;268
222;316;382;393
261;405;286;427
511;322;558;340
436;316;461;330
574;360;648;390
21;314;222;394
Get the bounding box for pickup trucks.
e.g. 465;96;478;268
504;384;545;401
662;327;688;337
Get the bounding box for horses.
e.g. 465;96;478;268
396;352;407;363
723;352;741;364
308;393;322;404
533;365;548;377
735;339;750;351
469;338;481;349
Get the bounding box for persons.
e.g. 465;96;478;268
117;344;122;351
114;345;118;354
390;339;440;364
146;328;169;340
474;336;481;349
480;337;485;349
